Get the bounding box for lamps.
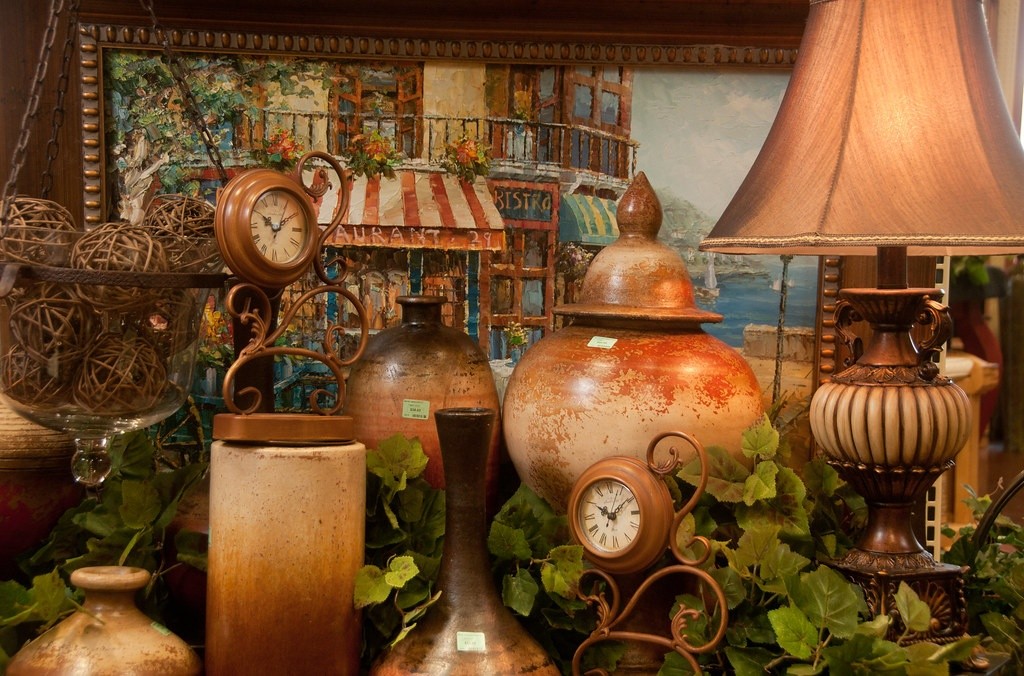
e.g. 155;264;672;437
701;0;1024;639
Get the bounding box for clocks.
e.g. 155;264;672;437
568;456;674;578
216;169;319;288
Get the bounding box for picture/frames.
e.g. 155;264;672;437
74;17;838;468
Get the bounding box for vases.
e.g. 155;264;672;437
373;408;558;676
347;293;501;490
4;564;201;676
